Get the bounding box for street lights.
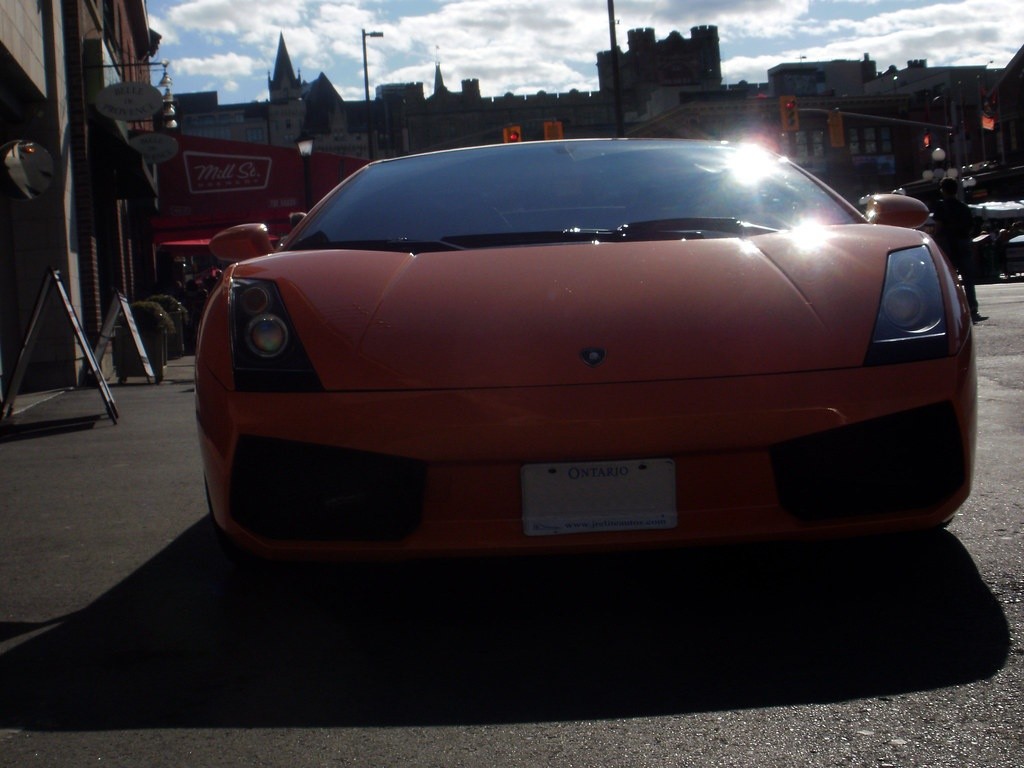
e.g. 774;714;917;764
294;129;316;212
361;28;383;159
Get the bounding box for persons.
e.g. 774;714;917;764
933;177;1024;325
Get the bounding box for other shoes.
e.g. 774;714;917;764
972;316;989;324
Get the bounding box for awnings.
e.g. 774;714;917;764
144;130;375;244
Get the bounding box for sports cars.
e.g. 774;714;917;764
194;137;978;581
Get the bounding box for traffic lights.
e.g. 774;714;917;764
920;132;934;148
779;95;800;133
503;125;522;144
